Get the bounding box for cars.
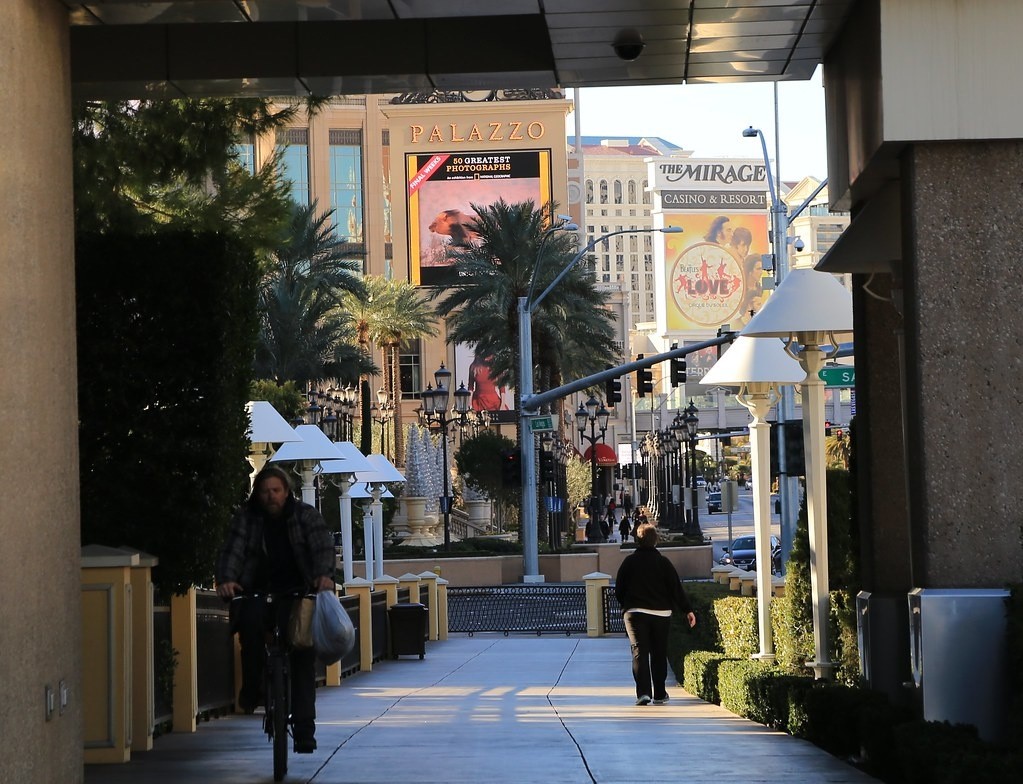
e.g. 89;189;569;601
705;492;733;515
719;534;781;578
744;477;753;491
690;476;708;486
704;478;732;494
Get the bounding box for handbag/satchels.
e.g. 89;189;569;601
308;590;355;664
291;594;318;647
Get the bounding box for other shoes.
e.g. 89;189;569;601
292;730;316;753
636;695;651;706
238;686;259;712
654;691;670;703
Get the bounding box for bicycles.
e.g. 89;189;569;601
222;585;315;784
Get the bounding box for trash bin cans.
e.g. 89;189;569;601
386;603;429;661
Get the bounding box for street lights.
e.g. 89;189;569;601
517;224;684;576
421;360;473;552
371;403;396;456
742;125;830;578
574;390;611;544
684;397;704;538
290;396;340;515
678;407;694;535
541;432;567;553
639;411;684;534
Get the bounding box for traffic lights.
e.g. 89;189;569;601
636;354;653;398
605;364;622;407
670;343;687;388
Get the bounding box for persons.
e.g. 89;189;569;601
214;468;336;750
686;347;716;367
615;524;696;705
707;480;712;494
704;217;763;331
467;342;509;411
585;493;647;542
